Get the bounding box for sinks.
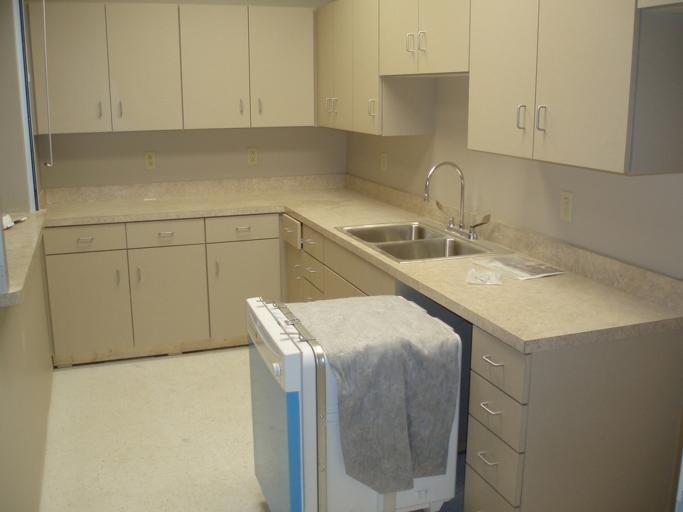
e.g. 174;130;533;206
368;238;494;264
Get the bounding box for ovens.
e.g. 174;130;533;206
245;296;462;511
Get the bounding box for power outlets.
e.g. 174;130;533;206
558;191;573;223
247;148;258;166
143;151;156;170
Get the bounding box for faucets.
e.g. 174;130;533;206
423;161;465;231
334;221;451;244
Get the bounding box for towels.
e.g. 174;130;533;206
283;296;461;494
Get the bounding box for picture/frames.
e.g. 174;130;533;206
203;213;281;350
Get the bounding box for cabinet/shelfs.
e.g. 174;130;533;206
352;0;382;136
41;218;211;367
378;0;471;77
462;324;533;512
282;213;396;302
466;0;642;176
